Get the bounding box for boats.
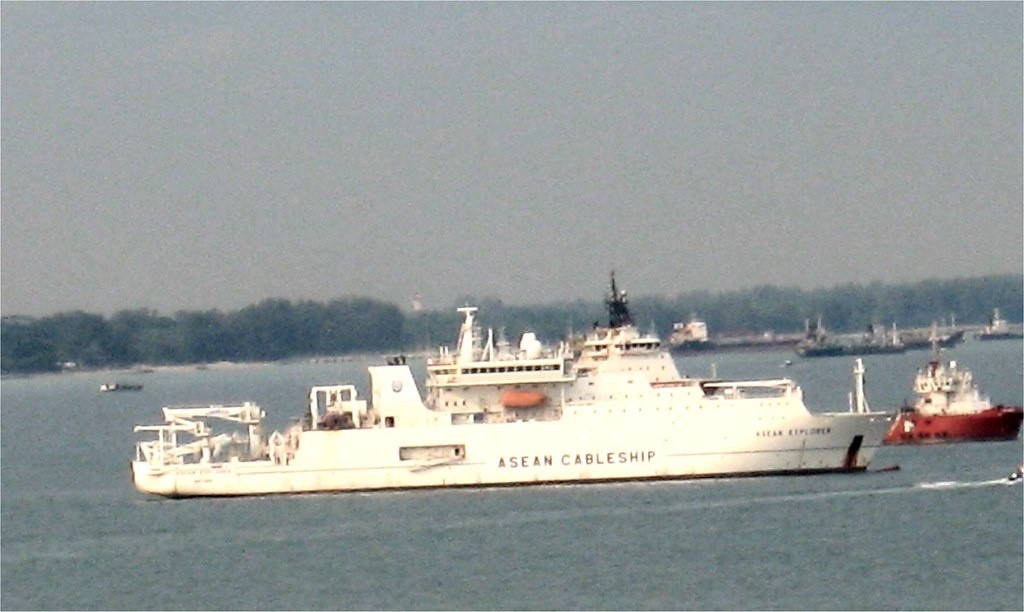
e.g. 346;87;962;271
899;319;966;347
100;384;142;392
794;315;906;356
976;308;1023;339
131;270;897;497
660;319;801;354
884;360;1024;445
